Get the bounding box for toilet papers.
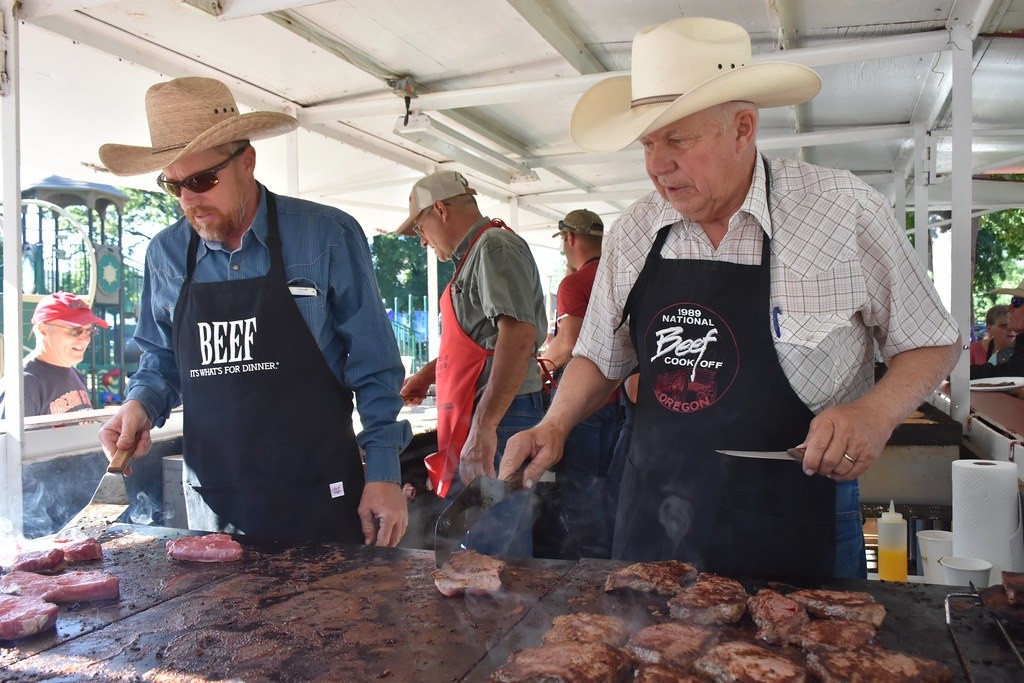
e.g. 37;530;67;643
951;459;1024;588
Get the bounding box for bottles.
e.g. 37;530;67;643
877;500;907;583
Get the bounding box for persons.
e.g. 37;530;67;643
534;209;623;559
399;170;548;557
98;77;414;547
497;17;963;579
0;291;109;420
946;278;1024;382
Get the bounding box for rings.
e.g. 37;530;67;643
843;453;855;464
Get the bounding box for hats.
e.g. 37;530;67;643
552;209;604;238
31;291;108;328
988;278;1024;297
570;16;821;153
398;170;478;236
99;77;300;176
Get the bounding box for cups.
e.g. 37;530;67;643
916;530;953;585
940;557;993;588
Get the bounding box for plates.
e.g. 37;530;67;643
970;377;1024;391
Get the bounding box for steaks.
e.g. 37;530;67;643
434;549;507;596
978;570;1024;626
485;555;955;683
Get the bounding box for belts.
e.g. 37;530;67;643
515;392;539;398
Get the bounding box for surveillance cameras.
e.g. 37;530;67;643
396;114;430;133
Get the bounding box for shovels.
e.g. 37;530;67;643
53;431;141;543
435;468;524;571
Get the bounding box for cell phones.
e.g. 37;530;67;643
288;286;317;298
451;283;461;295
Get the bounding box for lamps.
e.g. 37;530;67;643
395;112;539;185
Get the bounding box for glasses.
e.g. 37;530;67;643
46;323;92;337
157;142;250;197
1011;296;1024;307
559;221;575;231
413;203;451;239
991;323;1008;329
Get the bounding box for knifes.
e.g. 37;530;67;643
715;448;805;460
